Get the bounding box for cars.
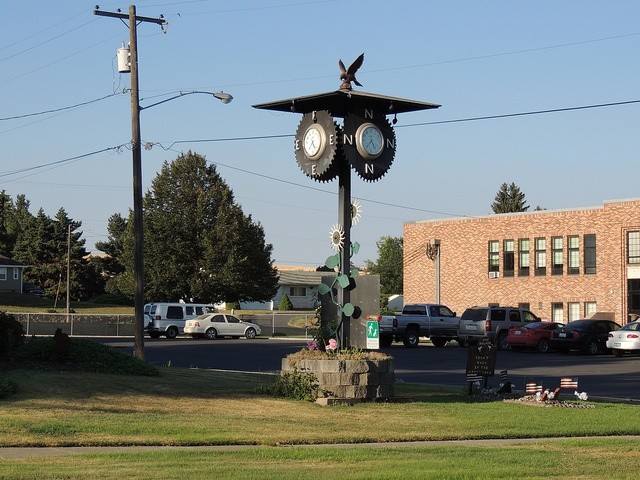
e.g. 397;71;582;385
183;312;262;339
506;321;565;352
549;319;622;355
605;322;640;357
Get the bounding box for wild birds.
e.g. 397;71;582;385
339;52;364;86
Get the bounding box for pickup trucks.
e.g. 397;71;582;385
366;303;462;348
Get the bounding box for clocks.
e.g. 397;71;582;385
301;123;326;160
355;122;385;160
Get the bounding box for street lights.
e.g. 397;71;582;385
130;90;234;359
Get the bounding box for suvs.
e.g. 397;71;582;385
457;305;541;351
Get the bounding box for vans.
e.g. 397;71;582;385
144;298;221;338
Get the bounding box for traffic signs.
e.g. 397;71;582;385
365;320;380;350
